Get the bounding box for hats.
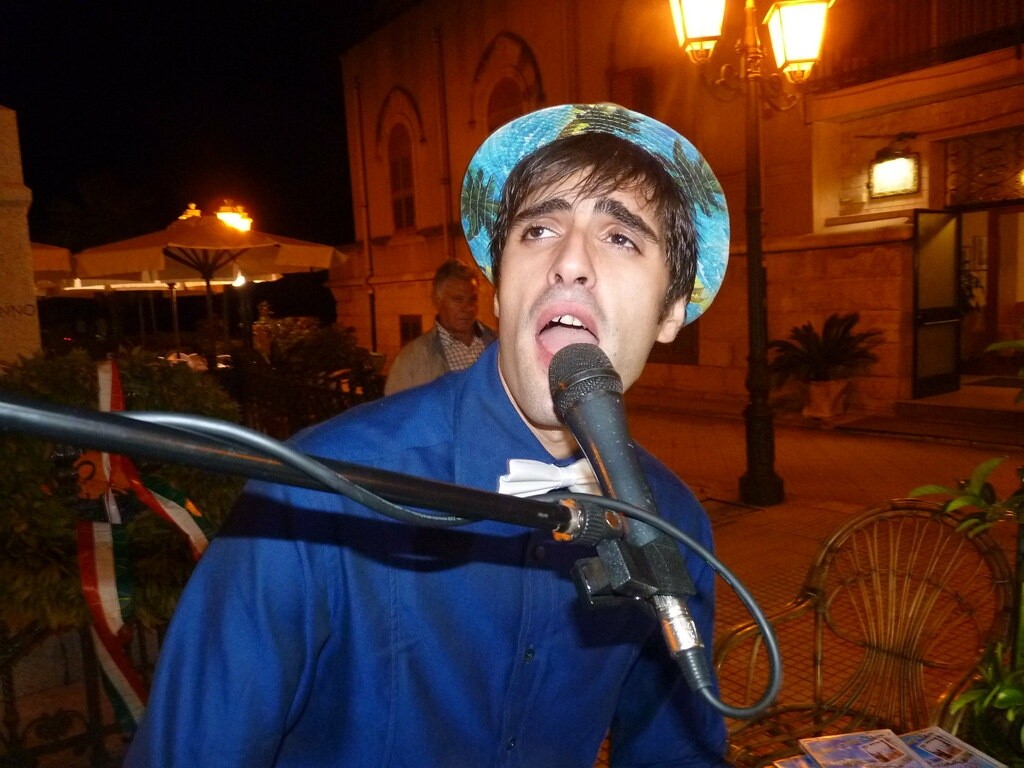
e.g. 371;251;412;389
460;101;730;328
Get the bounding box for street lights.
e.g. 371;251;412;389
670;1;839;510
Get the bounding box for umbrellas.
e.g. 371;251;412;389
27;206;347;368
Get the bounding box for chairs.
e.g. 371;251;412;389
710;500;1014;768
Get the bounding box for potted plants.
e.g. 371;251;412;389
770;312;885;419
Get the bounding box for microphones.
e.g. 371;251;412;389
549;344;712;693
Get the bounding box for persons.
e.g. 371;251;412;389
120;100;734;768
